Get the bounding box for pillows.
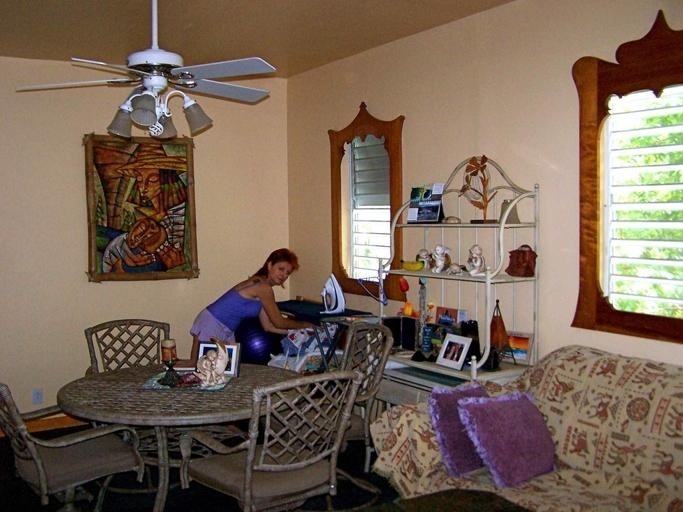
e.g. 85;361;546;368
429;380;488;476
453;389;559;491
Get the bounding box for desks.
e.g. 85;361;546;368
55;364;305;512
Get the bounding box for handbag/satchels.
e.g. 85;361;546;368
504;245;538;277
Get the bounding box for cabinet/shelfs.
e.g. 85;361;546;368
381;157;540;371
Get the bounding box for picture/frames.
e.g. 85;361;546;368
433;332;471;369
80;131;200;282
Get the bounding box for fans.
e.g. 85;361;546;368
12;0;276;139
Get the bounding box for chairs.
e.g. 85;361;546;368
271;315;393;473
177;363;363;510
0;380;148;511
81;320;169;372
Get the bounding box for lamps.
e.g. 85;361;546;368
102;84;213;141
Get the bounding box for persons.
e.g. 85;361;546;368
464;244;490;278
430;244;453;274
452;343;461;360
187;248;319;367
196;349;223;386
443;344;454;359
416;248;433;270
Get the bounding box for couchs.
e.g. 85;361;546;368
366;339;680;511
